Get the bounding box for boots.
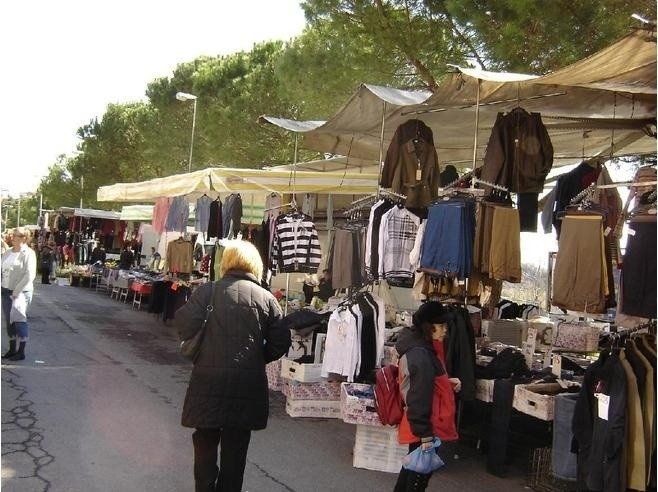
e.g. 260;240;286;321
11;342;25;360
5;339;16;358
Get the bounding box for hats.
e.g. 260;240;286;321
412;301;456;326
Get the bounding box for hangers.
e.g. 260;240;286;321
338;278;374;313
626;191;657;221
605;320;657;359
554;184;609;222
201;192;209;199
214;195;221;206
335;189;406;230
503;98;529;118
174;231;191;243
430;172;516;208
287;200;304;220
648;160;657;167
420;274;467;306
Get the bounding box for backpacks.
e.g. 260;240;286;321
373;364;404;426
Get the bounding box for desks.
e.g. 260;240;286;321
87;262;166;311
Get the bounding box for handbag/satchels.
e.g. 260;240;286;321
177;327;205;358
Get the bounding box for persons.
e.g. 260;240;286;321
42;240;56;285
89;243;107;265
318;268;336;303
174;239;291;492
1;226;37;361
117;244;136;270
392;300;463;491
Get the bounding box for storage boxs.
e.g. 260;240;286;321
474;377;496;404
281;357;410;473
550;393;580;482
512;379;582;422
